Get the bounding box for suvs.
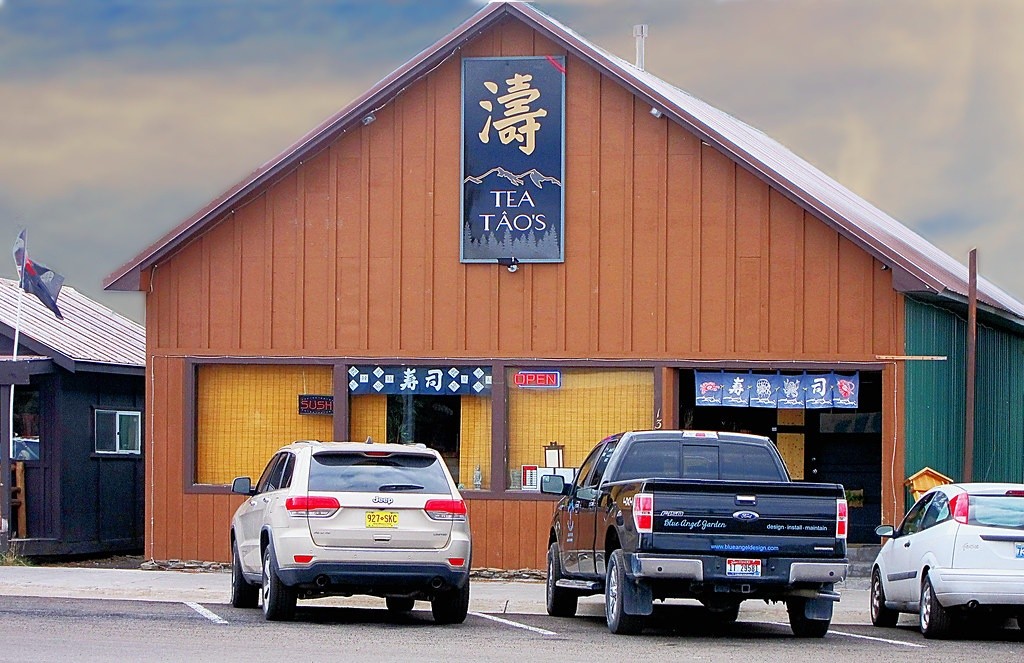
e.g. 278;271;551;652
227;434;473;624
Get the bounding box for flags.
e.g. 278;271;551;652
22;256;65;320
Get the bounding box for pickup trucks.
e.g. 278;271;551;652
544;429;849;639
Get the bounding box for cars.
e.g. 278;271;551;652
871;482;1024;638
